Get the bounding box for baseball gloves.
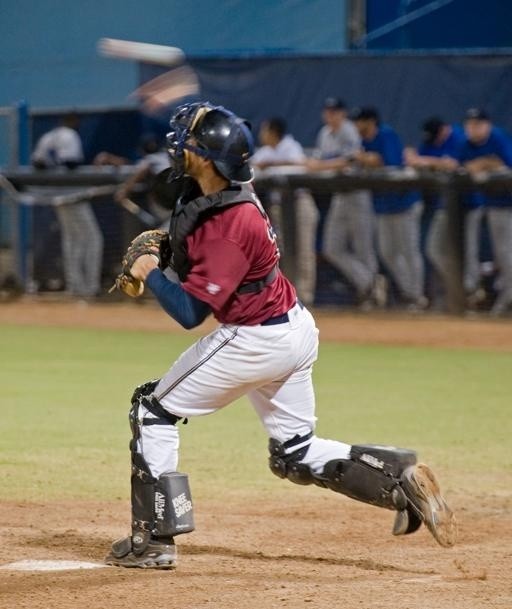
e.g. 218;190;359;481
108;229;173;298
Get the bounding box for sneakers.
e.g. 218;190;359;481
104;535;177;569
400;464;457;549
359;274;492;313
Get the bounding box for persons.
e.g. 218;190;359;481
103;99;459;569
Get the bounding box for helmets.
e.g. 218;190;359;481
164;102;255;184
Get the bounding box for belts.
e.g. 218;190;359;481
259;301;305;325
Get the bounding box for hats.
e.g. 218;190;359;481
421;116;437;143
318;96;373;120
464;105;485;118
